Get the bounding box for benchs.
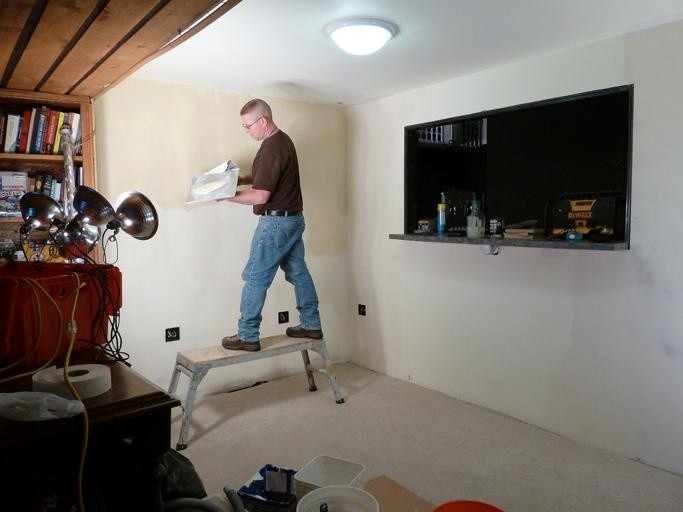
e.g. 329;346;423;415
167;332;344;452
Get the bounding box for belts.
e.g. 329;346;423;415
262;210;299;217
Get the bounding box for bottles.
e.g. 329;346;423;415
466;200;486;240
438;192;447;235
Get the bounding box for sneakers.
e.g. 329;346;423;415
286;326;323;339
222;335;260;351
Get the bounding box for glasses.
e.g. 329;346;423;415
242;118;261;129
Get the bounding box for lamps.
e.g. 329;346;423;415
322;18;399;57
20;185;159;240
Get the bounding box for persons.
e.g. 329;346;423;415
212;98;323;352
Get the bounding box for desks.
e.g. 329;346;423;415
363;474;435;512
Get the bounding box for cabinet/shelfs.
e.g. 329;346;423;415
0;345;181;512
0;88;100;263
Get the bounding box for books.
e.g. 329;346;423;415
0;103;83;225
